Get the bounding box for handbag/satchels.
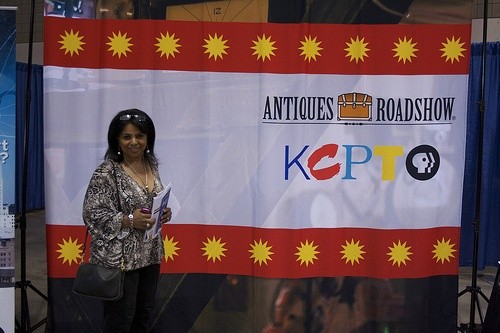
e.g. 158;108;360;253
72;263;126;300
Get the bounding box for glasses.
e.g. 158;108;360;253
120;114;146;121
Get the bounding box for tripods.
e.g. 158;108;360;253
458;0;491;333
15;0;48;333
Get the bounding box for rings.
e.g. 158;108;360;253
145;222;150;227
166;217;169;221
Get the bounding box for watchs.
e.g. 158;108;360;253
128;213;134;230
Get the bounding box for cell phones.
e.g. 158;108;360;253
141;207;151;214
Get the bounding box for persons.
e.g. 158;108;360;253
82;108;173;333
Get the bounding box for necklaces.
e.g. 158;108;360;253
125;159;150;190
